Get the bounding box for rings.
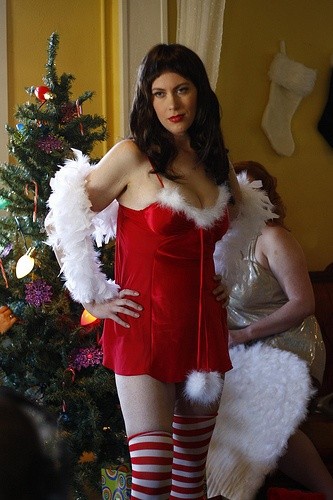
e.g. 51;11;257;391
9;315;13;319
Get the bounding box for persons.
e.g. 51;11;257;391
44;43;268;500
228;162;333;500
0;305;17;337
